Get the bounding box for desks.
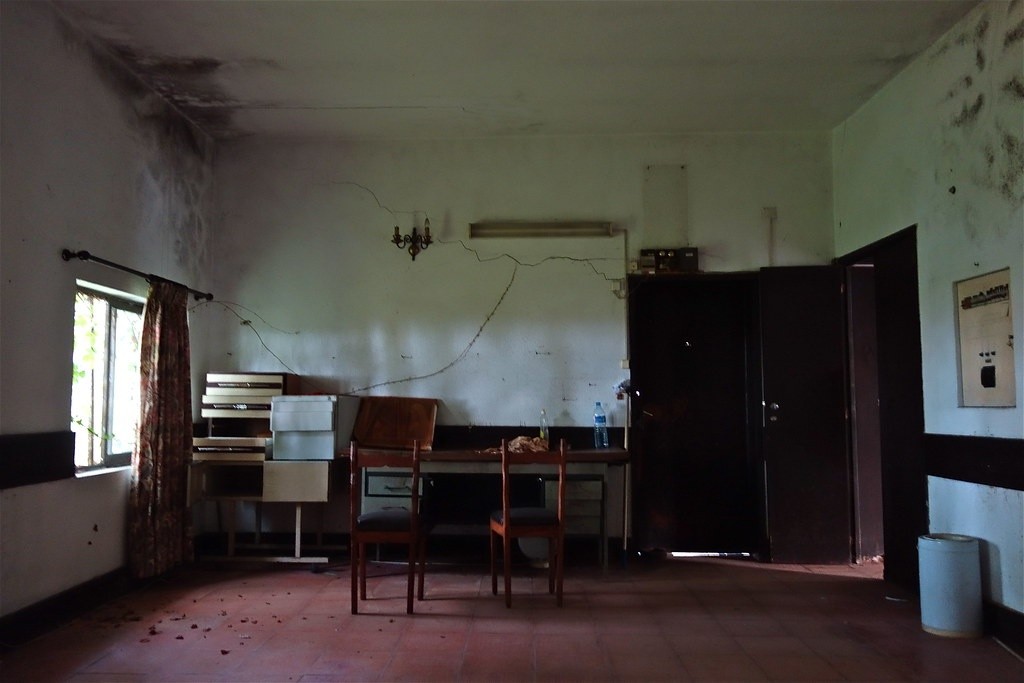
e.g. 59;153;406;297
339;445;630;564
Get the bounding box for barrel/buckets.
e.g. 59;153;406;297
917;532;984;638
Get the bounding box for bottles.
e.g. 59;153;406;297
592;401;608;448
539;408;549;440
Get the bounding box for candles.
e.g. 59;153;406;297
424;218;430;236
394;225;400;235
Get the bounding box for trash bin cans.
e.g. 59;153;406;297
917;533;987;639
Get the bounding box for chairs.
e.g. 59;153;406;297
490;439;567;609
348;439;431;616
